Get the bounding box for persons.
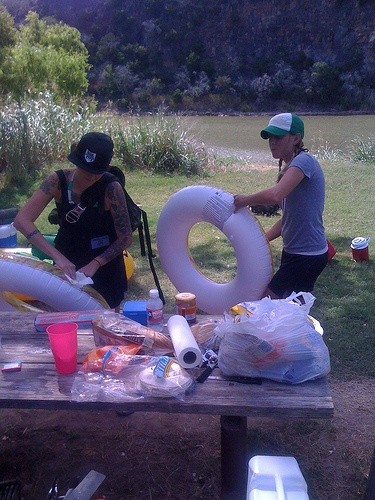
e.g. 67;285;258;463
233;112;329;299
13;132;133;415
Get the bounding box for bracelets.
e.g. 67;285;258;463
93;259;101;269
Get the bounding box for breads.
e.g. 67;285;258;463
138;328;174;350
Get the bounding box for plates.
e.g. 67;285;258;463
139;365;193;398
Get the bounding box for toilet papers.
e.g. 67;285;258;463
166;314;202;369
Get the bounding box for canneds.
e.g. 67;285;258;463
174;292;197;324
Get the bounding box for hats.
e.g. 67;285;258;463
260;112;305;139
67;131;114;173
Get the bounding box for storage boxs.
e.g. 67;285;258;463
122;300;148;326
0;223;17;248
34;309;101;332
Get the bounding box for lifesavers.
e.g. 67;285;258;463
157;185;274;314
0;249;112;312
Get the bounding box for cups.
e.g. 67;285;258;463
46;321;79;375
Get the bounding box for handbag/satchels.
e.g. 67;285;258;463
212;290;332;387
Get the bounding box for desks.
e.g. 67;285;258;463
0;310;335;500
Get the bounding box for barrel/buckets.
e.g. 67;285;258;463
351;237;371;263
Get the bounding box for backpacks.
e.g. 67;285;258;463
55;165;142;233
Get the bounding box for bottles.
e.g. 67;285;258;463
146;288;164;332
